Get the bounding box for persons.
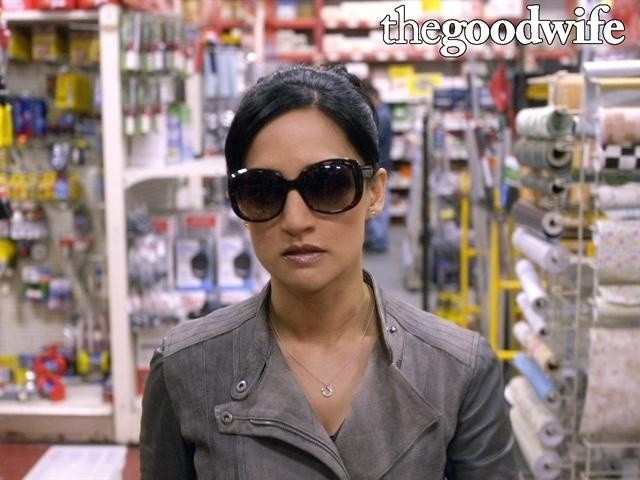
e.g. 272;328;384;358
138;61;538;480
359;79;393;254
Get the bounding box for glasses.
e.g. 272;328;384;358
228;159;380;222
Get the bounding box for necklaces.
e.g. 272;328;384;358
269;287;374;398
